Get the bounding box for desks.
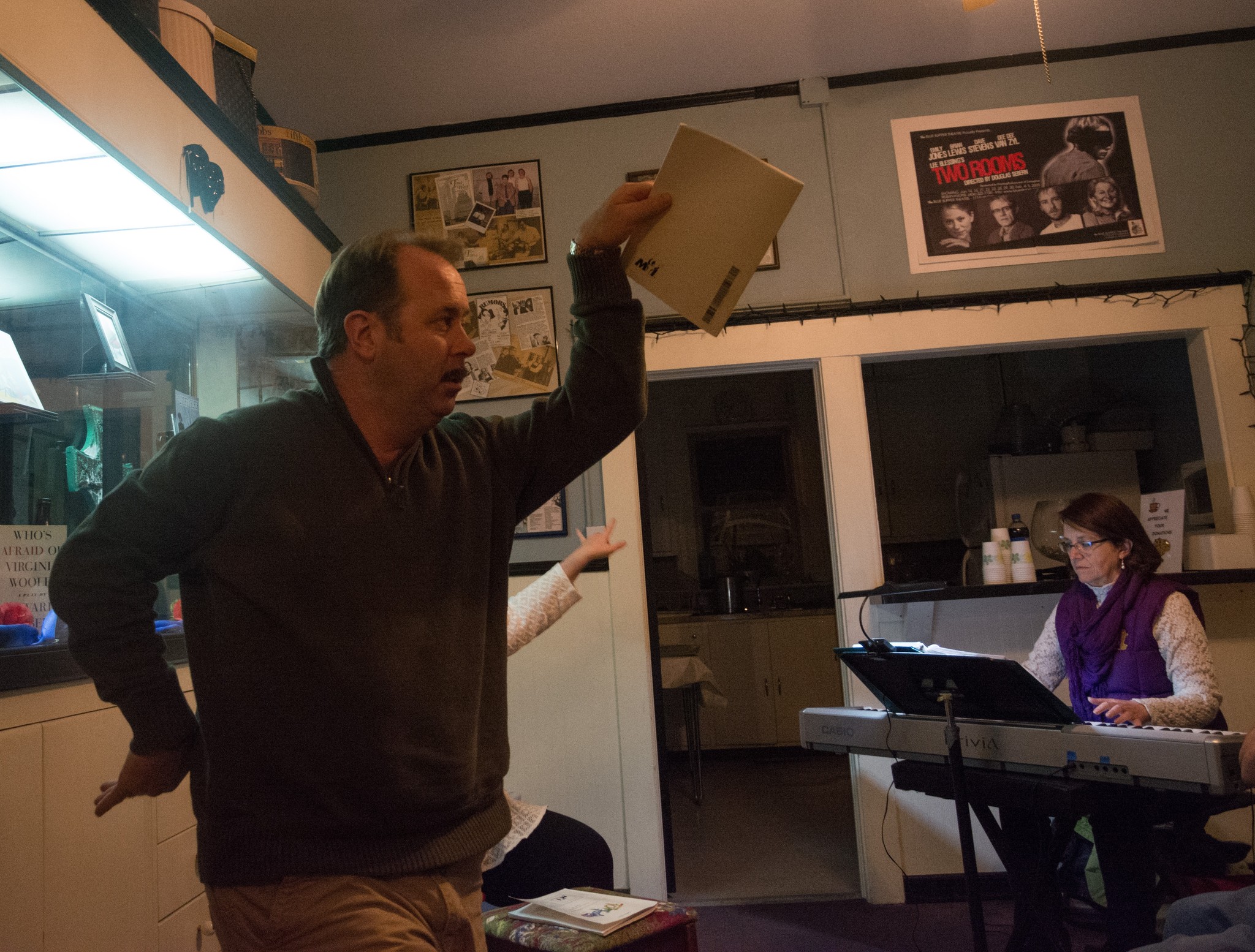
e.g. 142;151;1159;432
661;657;731;802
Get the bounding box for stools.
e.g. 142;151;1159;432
481;885;701;952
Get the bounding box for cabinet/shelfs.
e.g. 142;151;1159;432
0;370;156;423
662;614;843;750
0;664;216;952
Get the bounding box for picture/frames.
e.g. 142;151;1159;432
82;294;139;375
409;159;547;270
455;284;562;402
627;158;781;271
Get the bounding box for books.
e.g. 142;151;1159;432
508;888;658;937
616;123;808;338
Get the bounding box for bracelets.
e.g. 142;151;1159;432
569;237;622;257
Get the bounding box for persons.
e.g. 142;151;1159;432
479;169;533;215
1001;491;1229;952
531;333;552;348
514;298;533;315
938;115;1132;254
495;345;554;387
482;518;630;905
1125;730;1255;952
495;218;539;259
49;181;673;952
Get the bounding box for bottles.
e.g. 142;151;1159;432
1009;513;1030;540
33;498;52;526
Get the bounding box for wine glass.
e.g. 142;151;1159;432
1028;498;1084;590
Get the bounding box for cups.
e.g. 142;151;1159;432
980;526;1037;584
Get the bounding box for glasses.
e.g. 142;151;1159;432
992;206;1012;215
1057;538;1108;553
